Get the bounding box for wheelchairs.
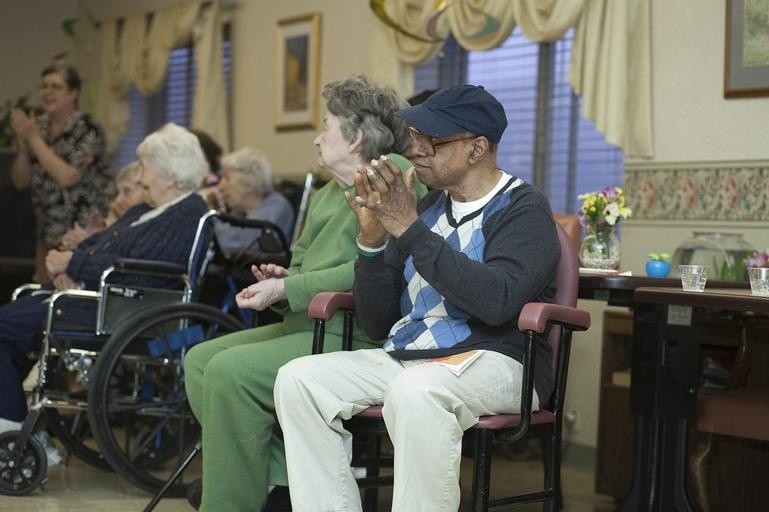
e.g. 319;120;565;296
0;212;295;497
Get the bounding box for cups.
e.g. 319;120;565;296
747;267;769;297
678;265;709;293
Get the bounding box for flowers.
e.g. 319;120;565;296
744;252;769;269
578;185;634;234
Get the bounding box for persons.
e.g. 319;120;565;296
206;145;295;328
57;159;142;253
272;85;562;512
2;122;210;486
189;123;227;215
184;77;429;511
8;60;113;286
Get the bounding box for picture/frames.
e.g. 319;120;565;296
271;10;325;132
722;0;769;101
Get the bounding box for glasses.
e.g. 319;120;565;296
408;126;478;157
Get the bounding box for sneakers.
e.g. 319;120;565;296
187;478;291;512
0;422;63;484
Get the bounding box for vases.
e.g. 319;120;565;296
578;237;622;269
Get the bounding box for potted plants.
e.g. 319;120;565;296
645;250;671;277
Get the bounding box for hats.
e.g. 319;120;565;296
395;84;509;143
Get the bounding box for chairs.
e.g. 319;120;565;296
308;215;591;511
